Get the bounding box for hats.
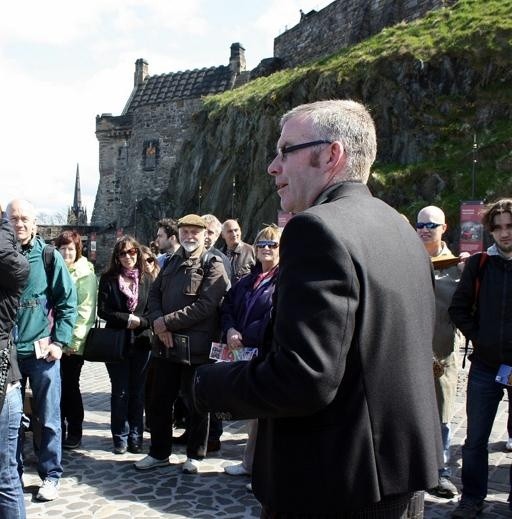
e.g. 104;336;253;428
178;214;207;228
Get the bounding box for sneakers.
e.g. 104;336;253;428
434;477;458;496
38;478;60;500
223;464;249;475
62;437;81;447
114;418;221;473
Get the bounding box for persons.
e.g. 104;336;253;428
5;200;78;501
448;198;512;518
416;206;470;496
1;199;31;519
505;390;512;450
53;231;98;447
97;215;282;489
176;97;446;519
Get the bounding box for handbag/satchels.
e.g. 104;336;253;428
134;329;153;371
84;328;124;362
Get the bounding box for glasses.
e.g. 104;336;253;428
416;223;444;228
146;258;154;263
255;240;279;248
116;248;138;258
277;140;332;160
